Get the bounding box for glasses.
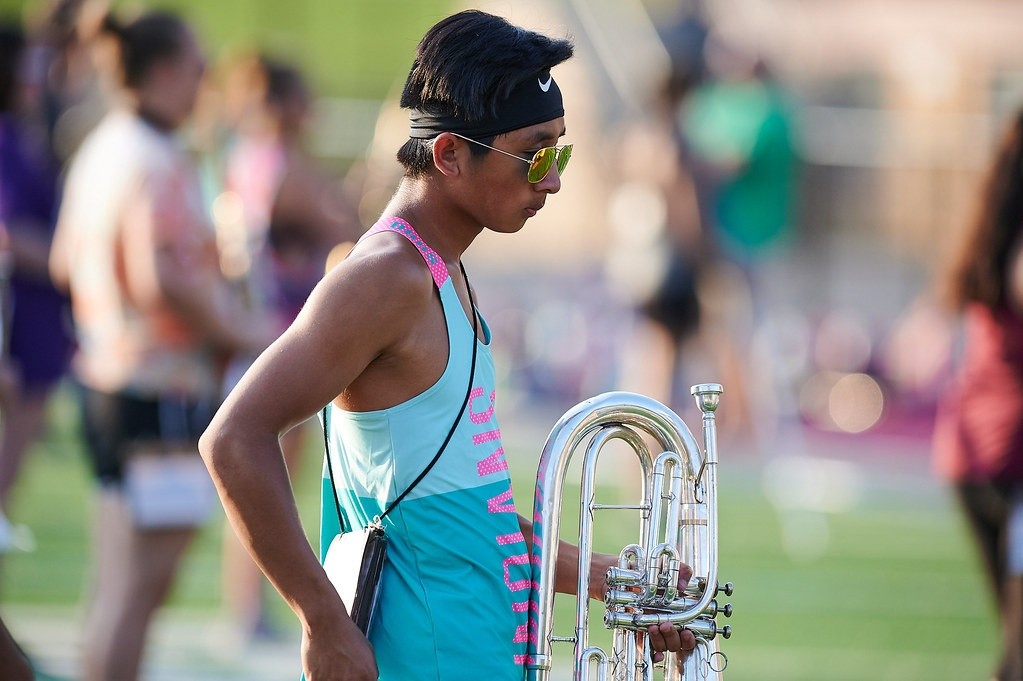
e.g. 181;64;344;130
441;131;572;185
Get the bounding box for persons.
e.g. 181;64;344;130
218;49;366;647
927;100;1023;680
48;9;268;680
198;9;578;681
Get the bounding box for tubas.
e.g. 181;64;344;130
520;381;736;681
